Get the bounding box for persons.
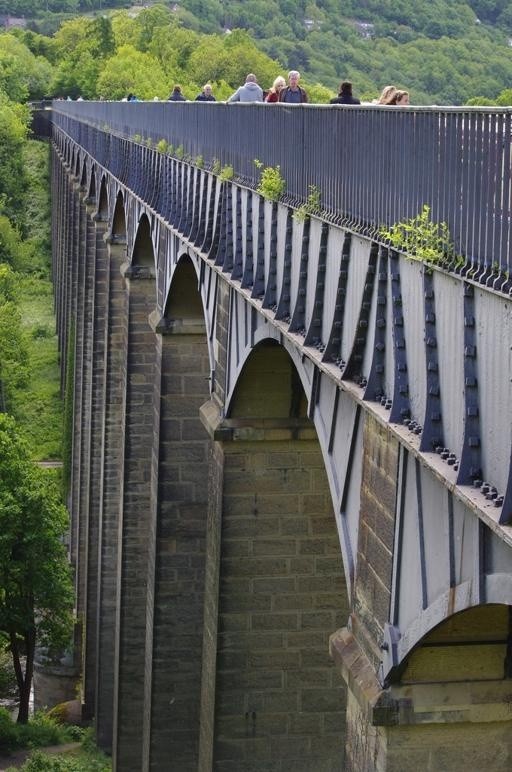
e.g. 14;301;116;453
194;83;215;101
376;85;396;104
387;89;410;105
277;70;308;102
265;74;286;102
329;80;360;103
226;73;263;101
168;85;186;99
